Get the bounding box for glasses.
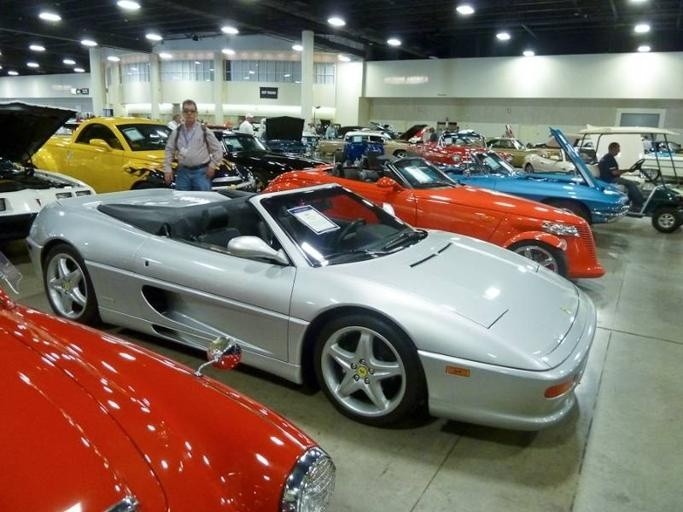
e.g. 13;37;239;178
183;110;196;113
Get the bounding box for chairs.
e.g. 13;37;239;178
190;201;299;252
332;159;380;182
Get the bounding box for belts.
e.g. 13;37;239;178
183;160;211;169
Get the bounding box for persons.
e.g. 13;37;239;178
597;141;645;213
166;112;438;145
162;100;226;191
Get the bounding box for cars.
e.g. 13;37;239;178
0;101;98;239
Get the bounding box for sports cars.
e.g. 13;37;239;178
27;116;257;196
25;184;596;432
265;121;682;234
0;285;336;512
259;152;607;285
209;130;327;191
